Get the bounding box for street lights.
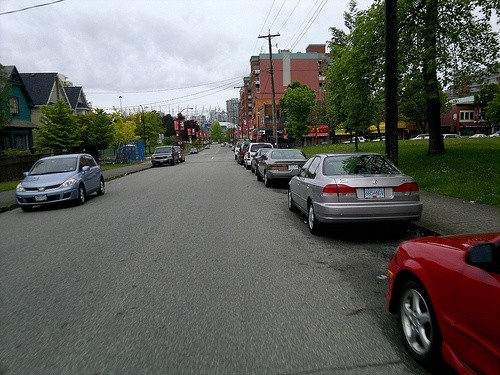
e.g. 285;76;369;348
178;107;193;148
139;105;148;157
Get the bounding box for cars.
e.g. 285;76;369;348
386;231;500;375
150;145;185;168
409;134;430;140
470;134;487;138
288;153;423;237
256;149;311;188
342;136;365;143
230;140;274;176
15;153;105;212
190;147;198;154
489;131;500;137
371;136;386;141
443;134;458;139
